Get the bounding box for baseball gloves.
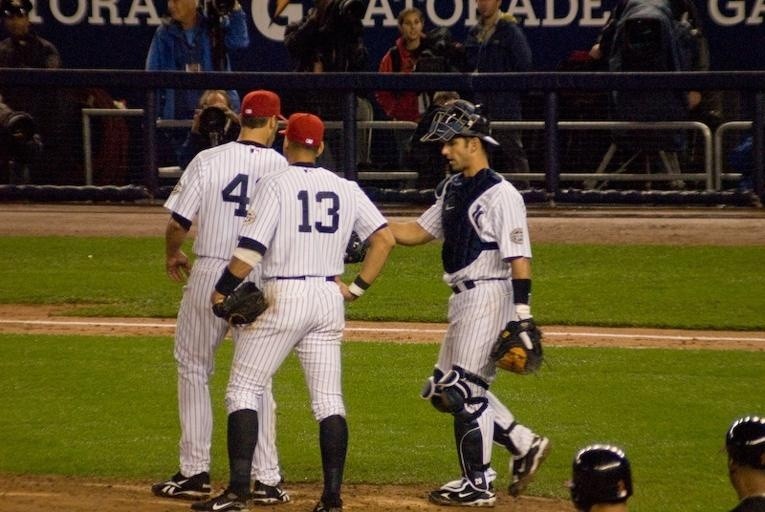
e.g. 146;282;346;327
212;281;267;324
490;317;543;375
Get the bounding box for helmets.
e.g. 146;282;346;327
418;103;503;149
725;415;764;468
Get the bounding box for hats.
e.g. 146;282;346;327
280;111;324;151
241;89;286;121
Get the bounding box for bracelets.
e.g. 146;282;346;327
512;279;532;304
214;266;243;297
348;275;370;298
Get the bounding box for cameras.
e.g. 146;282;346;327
198;107;226;132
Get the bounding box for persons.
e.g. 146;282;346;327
151;91;291;505
384;135;552;507
724;416;765;512
189;111;396;511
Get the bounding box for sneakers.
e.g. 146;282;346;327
190;489;250;511
151;471;211;501
312;500;343;512
252;481;291;506
508;436;552;499
431;482;496;508
